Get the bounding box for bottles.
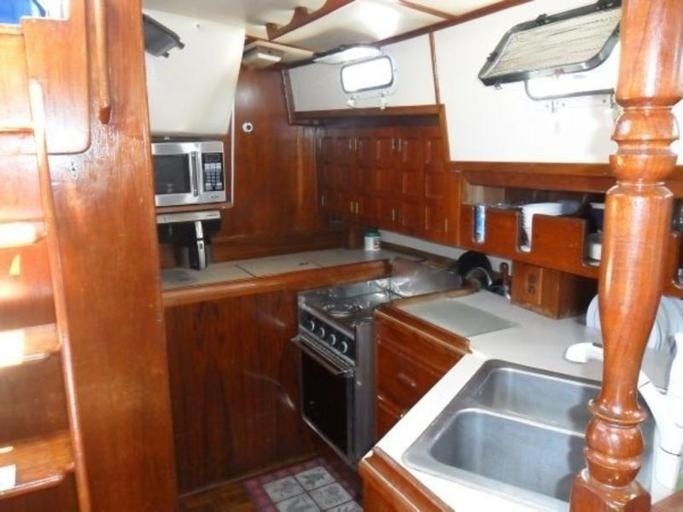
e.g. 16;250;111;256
364;228;381;252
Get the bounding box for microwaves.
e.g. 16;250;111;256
151;140;227;208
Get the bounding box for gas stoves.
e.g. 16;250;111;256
299;274;462;336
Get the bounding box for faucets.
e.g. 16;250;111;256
565;340;669;425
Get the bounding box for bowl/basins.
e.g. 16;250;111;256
522;200;583;246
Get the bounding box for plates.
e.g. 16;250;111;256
585;294;682;352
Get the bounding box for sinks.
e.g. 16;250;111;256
463;360;603;438
404;407;584;509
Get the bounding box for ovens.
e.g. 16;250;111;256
290;304;371;472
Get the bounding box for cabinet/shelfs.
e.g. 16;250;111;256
460;205;683;300
313;126;461;249
372;304;470;444
0;77;90;511
